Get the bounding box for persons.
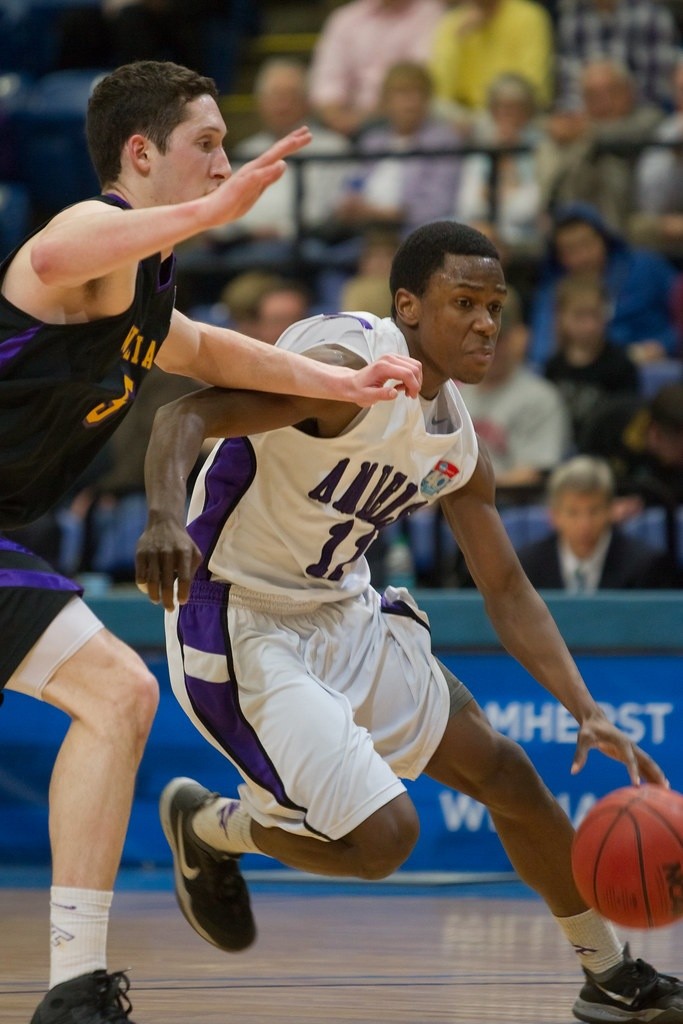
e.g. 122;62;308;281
0;0;680;591
130;224;683;1024
1;60;424;1024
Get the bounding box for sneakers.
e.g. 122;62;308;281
572;941;683;1024
31;966;136;1024
160;776;257;954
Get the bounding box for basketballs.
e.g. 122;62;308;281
570;784;683;930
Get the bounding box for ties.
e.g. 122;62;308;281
575;570;589;593
